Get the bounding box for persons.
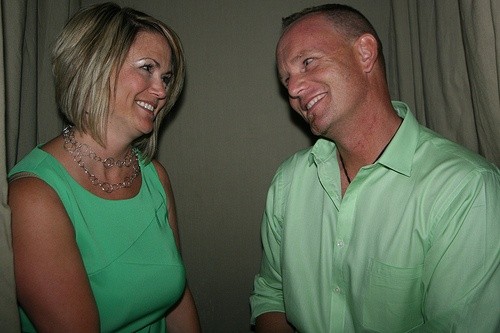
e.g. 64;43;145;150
248;4;500;333
7;2;201;333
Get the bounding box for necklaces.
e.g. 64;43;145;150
338;117;404;184
62;124;141;193
